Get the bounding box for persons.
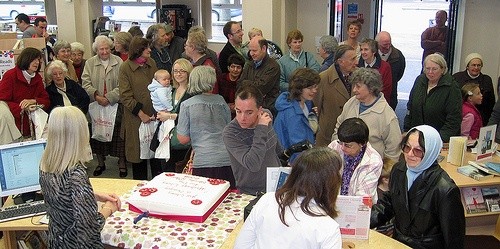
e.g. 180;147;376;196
276;30;320;93
118;36;163;180
236;36;280;124
31;17;56;62
15;13;36;39
330;67;402;186
0;100;23;146
184;32;220;94
487;75;500;145
358;38;393;106
218;54;245;120
114;32;132;61
318;35;338;73
273;68;321;167
44;39;82;87
0;47;51;139
338;20;363;68
39;105;121;249
149;23;177;75
177;64;235;190
233;145;343;249
374;30;406;111
156;58;195;172
128;26;144;39
246;28;283;61
371;124;465;249
460;83;483;140
314;45;360;148
181;25;220;63
403;54;462;143
46;60;90;115
219;21;250;74
147;68;174;123
420;10;448;74
452;53;495;127
80;35;128;177
146;26;154;46
70;42;88;83
222;84;284;197
165;25;186;65
327;117;383;206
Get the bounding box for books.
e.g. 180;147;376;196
16;230;48;249
447;136;468;166
495;150;500;156
461;187;487;214
471;142;498;153
457;164;494;181
486;161;500;176
481;187;500;212
468;160;500;176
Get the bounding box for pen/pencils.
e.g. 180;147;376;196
134;212;146;224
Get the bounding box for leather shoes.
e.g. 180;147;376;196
119;168;127;177
93;163;105;176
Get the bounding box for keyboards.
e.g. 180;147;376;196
0;199;47;229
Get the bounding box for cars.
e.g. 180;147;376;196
147;5;242;24
0;4;41;20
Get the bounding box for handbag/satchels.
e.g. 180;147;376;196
15;109;36;143
88;101;118;142
139;117;162;159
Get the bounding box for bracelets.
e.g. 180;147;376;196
102;206;113;216
170;115;172;119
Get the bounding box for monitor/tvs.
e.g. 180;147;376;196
0;139;47;205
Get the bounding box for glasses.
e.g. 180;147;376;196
173;69;187;74
153;48;170;64
231;29;244;35
401;143;424;158
35;25;47;29
184;44;191;47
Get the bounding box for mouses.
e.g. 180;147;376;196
46;214;49;219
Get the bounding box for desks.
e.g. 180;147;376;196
384;148;500;241
0;177;413;249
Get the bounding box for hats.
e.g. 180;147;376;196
465;52;483;67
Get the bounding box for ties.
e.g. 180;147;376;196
343;76;351;96
